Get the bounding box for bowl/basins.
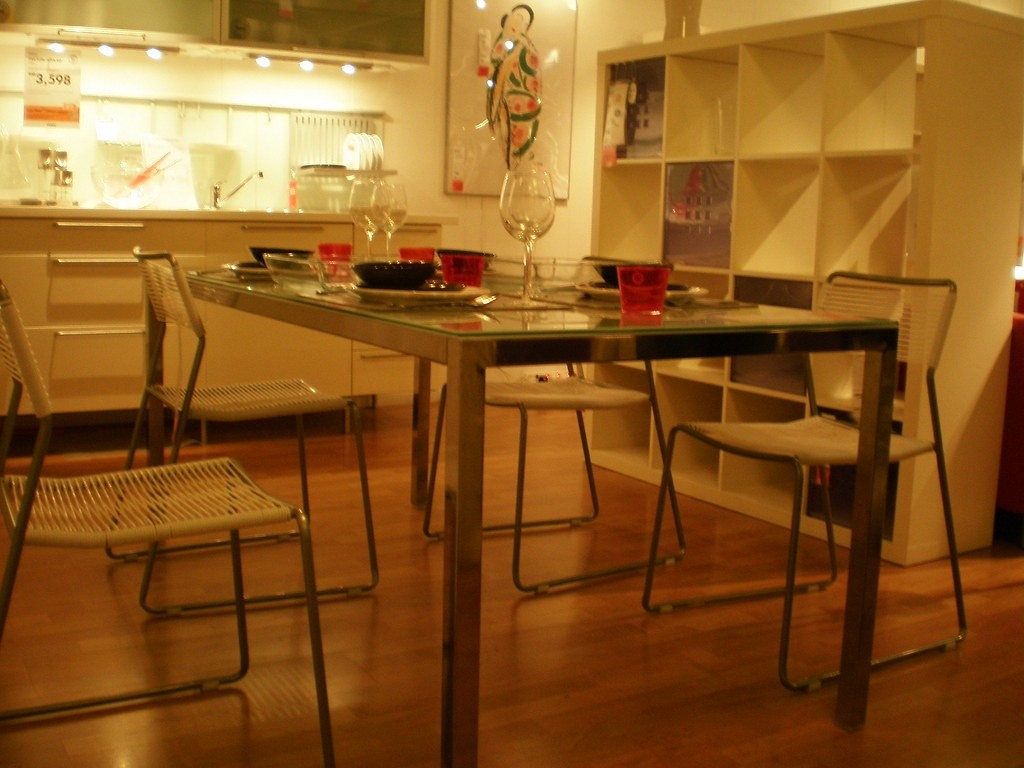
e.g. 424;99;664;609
437;249;496;259
249;247;314;268
349;263;438;291
224;262;273;281
594;265;619;289
263;253;363;301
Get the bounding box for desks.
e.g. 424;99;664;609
122;270;903;766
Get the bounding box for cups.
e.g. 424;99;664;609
441;254;485;287
617;266;670;327
399;247;435;263
318;244;353;262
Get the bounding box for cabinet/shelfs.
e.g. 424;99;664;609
574;0;1024;566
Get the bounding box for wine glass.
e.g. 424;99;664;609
349;182;379;263
500;170;555;309
372;183;407;264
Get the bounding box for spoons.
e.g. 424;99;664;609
402;292;502;307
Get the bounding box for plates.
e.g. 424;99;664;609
350;289;491;306
576;283;709;308
343;133;384;171
300;165;346;170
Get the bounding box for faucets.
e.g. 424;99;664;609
211;167;264;209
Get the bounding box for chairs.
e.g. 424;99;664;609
3;283;341;766
107;240;384;621
418;253;698;594
645;270;969;681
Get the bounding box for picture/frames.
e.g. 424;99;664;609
444;0;582;199
